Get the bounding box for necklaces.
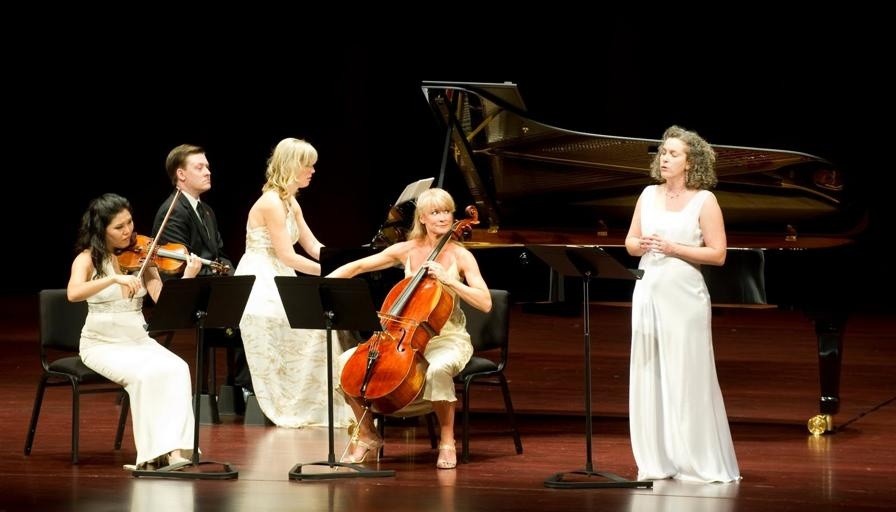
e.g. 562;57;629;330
667;194;679;199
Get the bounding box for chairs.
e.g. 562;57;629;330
24;285;133;464
376;286;525;463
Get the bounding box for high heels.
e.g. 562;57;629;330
341;433;383;465
436;439;458;470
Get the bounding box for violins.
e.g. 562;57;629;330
121;231;229;275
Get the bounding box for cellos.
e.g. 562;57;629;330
340;205;479;413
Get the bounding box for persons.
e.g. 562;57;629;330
323;188;492;469
151;144;255;417
234;137;377;429
624;125;743;486
66;193;202;472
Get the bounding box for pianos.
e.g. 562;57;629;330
320;79;852;436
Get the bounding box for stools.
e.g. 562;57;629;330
149;328;239;423
